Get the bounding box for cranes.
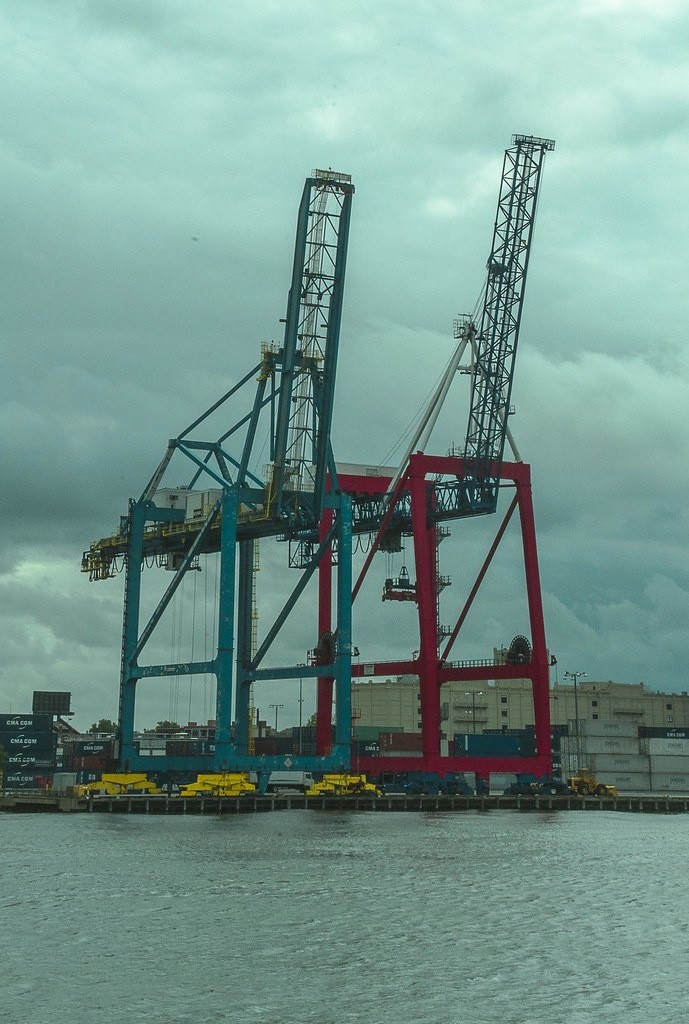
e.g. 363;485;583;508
81;169;355;583
276;134;557;569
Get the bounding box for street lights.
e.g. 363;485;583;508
296;664;305;755
563;671;588;779
464;691;482;734
268;704;284;732
464;711;472;733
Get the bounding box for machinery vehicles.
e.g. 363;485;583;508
568;768;618;797
